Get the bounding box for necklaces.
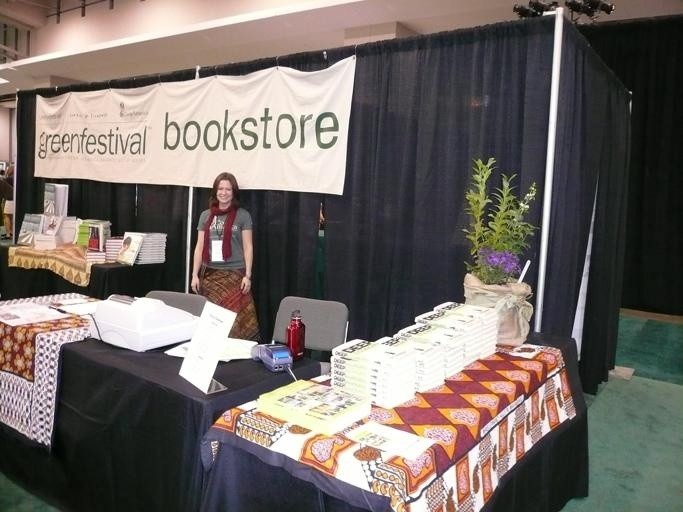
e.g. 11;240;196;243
215;214;229;239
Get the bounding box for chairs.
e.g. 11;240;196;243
268;296;350;365
145;291;207;317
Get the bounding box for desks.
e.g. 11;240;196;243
199;332;589;512
0;271;329;511
0;240;169;299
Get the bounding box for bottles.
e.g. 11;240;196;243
284;308;306;360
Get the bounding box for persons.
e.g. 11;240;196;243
0;170;5;176
191;172;260;343
0;167;14;240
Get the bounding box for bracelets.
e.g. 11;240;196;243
245;275;251;280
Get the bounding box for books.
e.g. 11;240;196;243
0;293;256;362
258;380;369;436
332;300;500;409
42;183;69;235
17;214;167;266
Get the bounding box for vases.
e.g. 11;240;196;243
463;274;533;349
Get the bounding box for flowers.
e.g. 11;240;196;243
464;154;540;284
